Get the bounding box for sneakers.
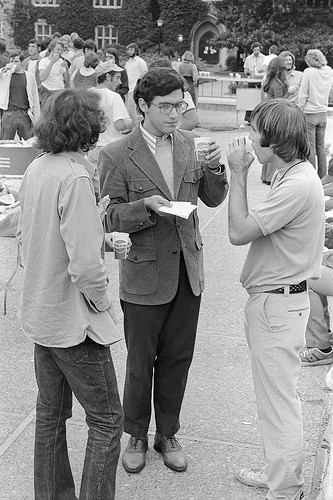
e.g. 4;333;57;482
297;347;333;367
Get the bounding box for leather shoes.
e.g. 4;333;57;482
121;435;149;473
153;430;188;472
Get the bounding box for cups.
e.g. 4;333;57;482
193;137;210;162
111;232;129;260
244;135;255;154
10;65;16;72
230;73;241;80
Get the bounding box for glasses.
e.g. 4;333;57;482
151;101;189;115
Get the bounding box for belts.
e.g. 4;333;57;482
264;280;307;294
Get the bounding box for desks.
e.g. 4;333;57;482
0;231;24;316
197;76;264;100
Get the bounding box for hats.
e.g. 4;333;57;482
95;59;125;78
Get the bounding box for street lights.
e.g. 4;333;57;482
156;17;166;56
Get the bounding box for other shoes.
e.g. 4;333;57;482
234;468;306;500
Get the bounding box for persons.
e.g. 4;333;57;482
225;98;326;500
243;42;333;367
0;32;201;203
18;86;132;500
97;68;230;473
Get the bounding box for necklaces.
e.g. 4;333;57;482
279;160;305;181
